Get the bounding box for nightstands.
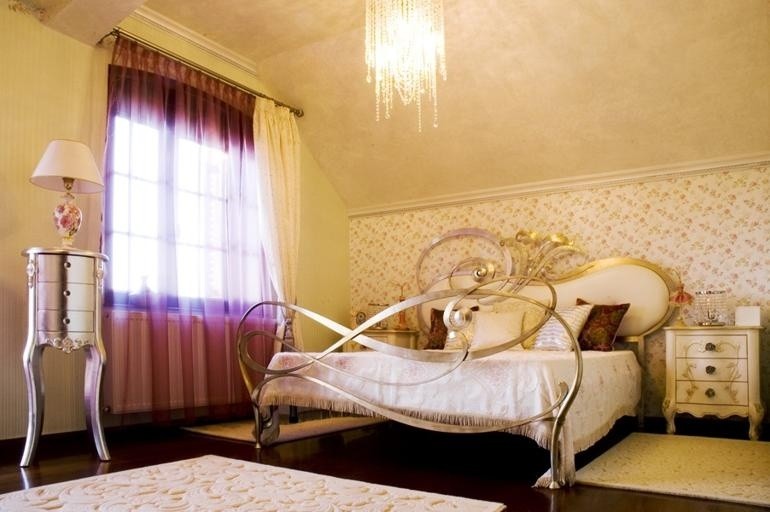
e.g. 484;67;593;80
664;327;764;442
342;330;419;352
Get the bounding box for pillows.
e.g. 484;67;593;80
535;305;593;350
443;311;476;353
424;306;477;350
468;311;525;350
493;300;551;350
576;299;631;352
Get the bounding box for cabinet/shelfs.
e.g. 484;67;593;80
20;247;108;463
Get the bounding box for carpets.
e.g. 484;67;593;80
572;431;770;507
179;410;392;447
0;454;506;511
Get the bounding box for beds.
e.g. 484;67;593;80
232;227;682;488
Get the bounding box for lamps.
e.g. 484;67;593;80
28;138;104;246
695;290;728;325
366;1;447;131
